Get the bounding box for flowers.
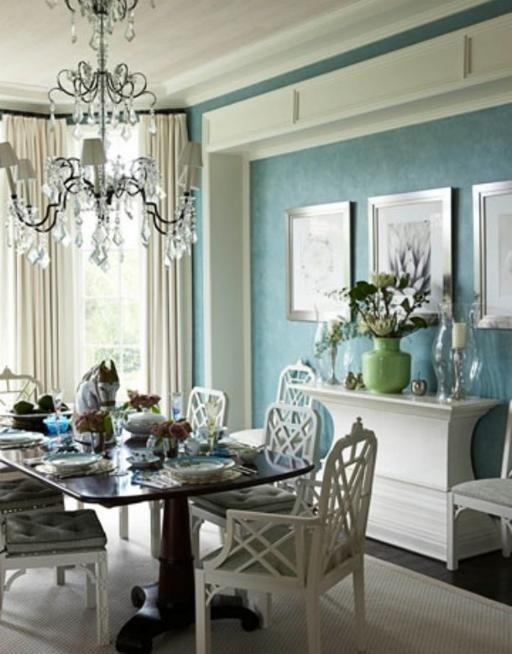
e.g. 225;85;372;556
312;267;444;356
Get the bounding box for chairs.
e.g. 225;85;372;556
446;398;512;572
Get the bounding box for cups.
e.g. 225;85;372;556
238;448;258;464
131;448;150;460
44;416;70;448
146;390;228;456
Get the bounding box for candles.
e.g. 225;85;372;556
452;318;467;351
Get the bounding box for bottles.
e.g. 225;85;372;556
313;298;357;384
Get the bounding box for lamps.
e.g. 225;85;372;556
0;1;205;270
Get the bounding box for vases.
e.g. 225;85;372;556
312;292;353;385
362;334;414;393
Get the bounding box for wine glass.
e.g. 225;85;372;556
51;389;67;445
108;409;130;476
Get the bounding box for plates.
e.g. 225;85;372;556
44;452;103;470
75;437;118;444
1;430;45;441
165;455;236;477
126;455;160;466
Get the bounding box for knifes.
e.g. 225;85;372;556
63;466;117;476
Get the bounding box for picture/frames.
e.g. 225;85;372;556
362;184;456;327
472;177;512;333
282;199;356;327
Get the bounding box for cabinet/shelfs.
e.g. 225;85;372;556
286;380;507;576
